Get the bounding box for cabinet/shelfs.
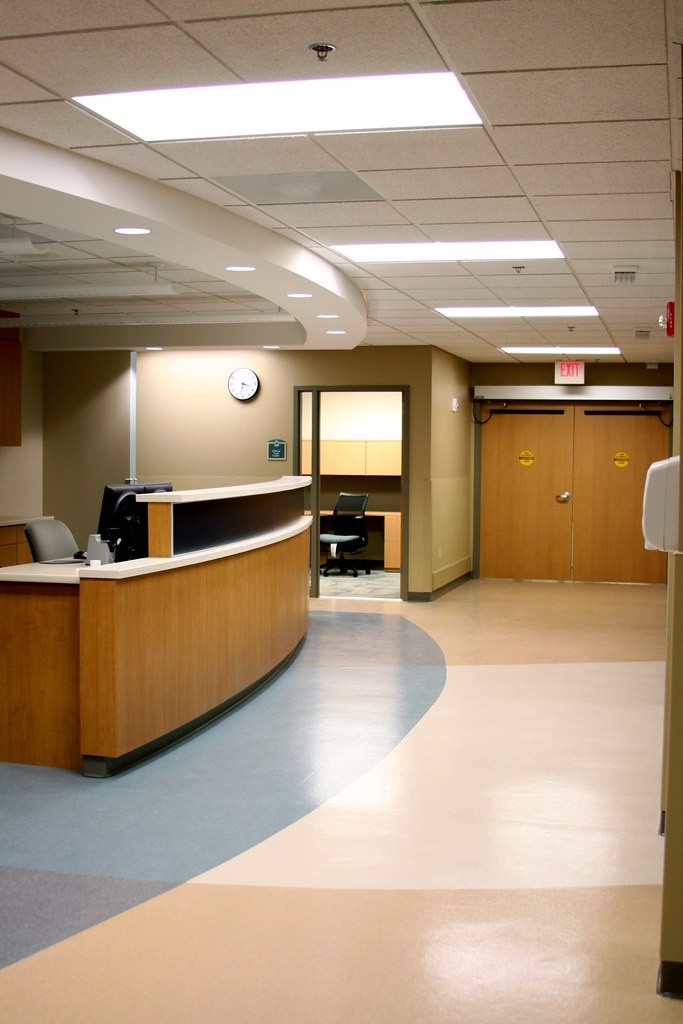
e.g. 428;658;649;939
302;440;401;476
0;514;56;568
304;510;402;572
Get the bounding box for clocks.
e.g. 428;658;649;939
228;367;259;400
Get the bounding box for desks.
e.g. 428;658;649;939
0;553;88;766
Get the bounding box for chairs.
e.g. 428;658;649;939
319;492;369;577
25;519;79;563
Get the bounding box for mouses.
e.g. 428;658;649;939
73;551;87;560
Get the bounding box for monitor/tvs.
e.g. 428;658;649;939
98;482;173;563
